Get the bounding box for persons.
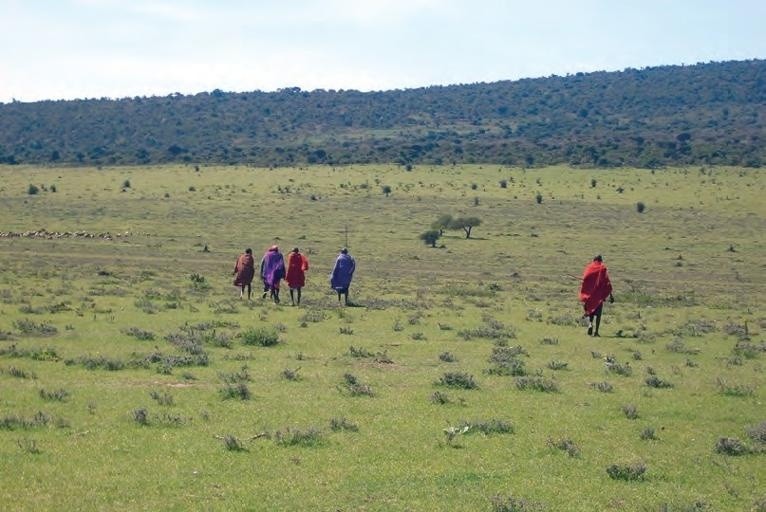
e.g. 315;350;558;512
580;255;615;337
262;245;286;303
232;248;254;300
259;248;274;299
331;247;355;305
285;247;305;307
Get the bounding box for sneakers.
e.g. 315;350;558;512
588;325;593;335
263;292;267;298
